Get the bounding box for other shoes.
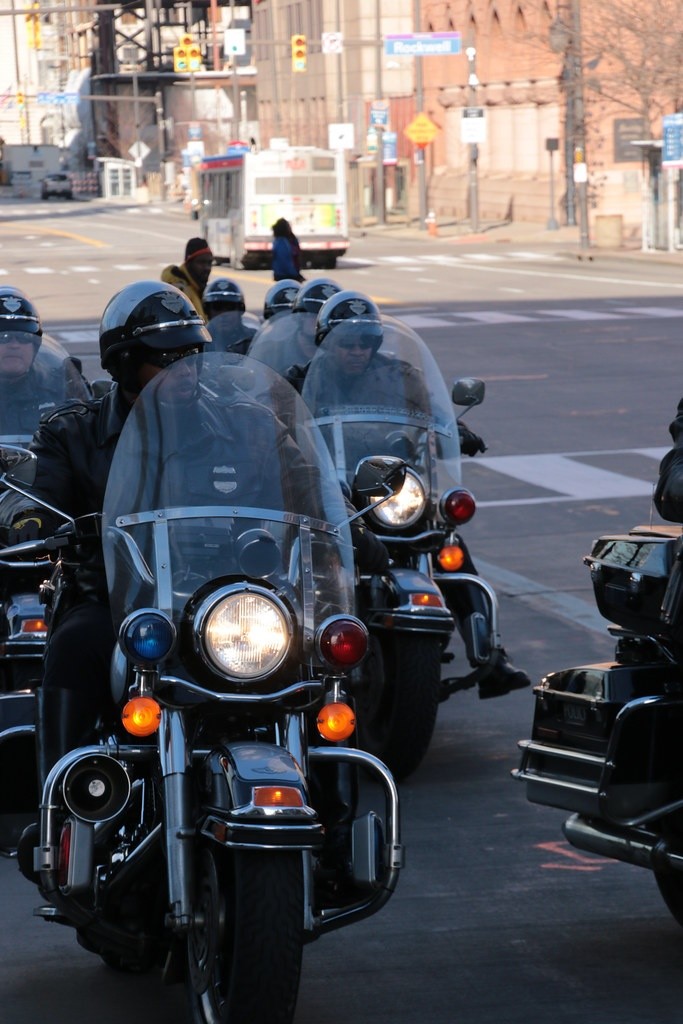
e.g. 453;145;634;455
18;822;56;886
321;801;354;864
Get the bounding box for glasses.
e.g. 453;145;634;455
140;348;199;368
0;331;36;344
336;335;377;350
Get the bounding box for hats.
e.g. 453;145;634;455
184;238;211;263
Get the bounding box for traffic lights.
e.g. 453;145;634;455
173;34;202;73
291;33;308;73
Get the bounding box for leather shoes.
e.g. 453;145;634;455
478;655;531;699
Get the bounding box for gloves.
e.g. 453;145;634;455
330;524;390;575
456;424;485;458
7;510;60;562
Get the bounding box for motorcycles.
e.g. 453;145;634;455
0;307;498;1024
510;526;683;925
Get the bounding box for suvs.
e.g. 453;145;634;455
38;173;73;199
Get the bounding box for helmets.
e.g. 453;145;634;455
314;290;384;354
99;279;213;370
201;279;246;315
0;284;42;350
263;278;344;323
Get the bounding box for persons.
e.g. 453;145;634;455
652;396;683;525
0;219;530;884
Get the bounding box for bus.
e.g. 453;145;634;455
193;139;352;274
